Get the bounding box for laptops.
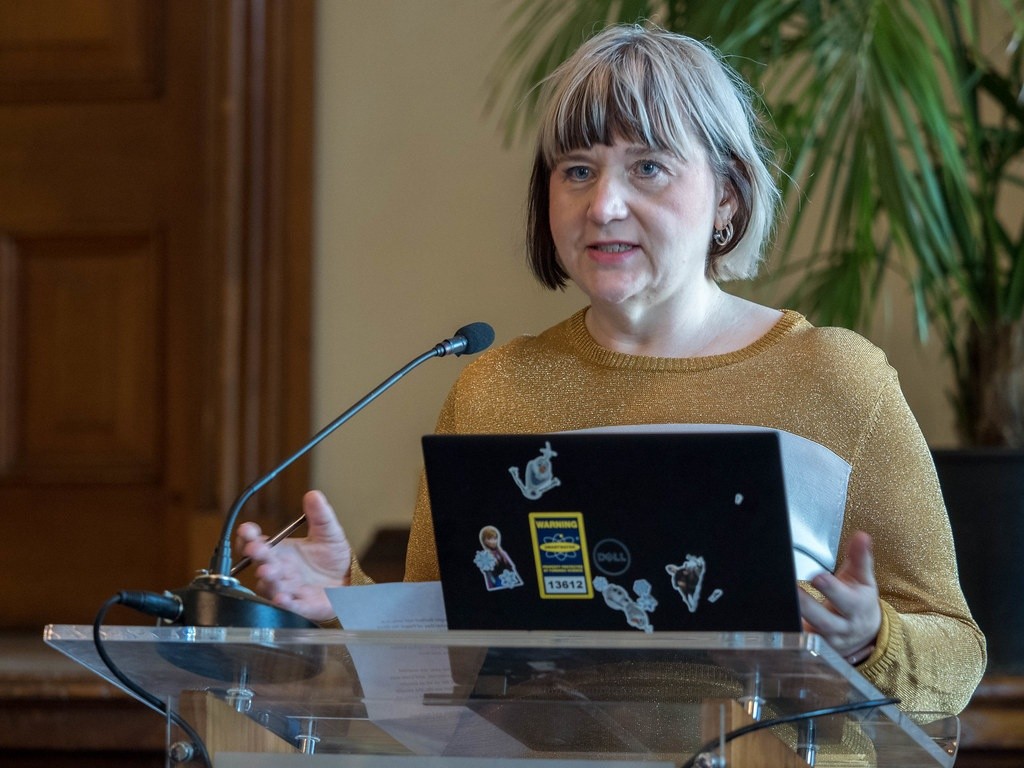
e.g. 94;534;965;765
416;431;858;755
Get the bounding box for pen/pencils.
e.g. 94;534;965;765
229;513;307;577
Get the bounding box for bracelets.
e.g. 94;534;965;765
839;642;877;668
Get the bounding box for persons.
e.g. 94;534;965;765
231;23;989;768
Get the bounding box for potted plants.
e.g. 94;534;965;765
477;0;1024;676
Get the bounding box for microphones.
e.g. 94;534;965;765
155;322;495;683
